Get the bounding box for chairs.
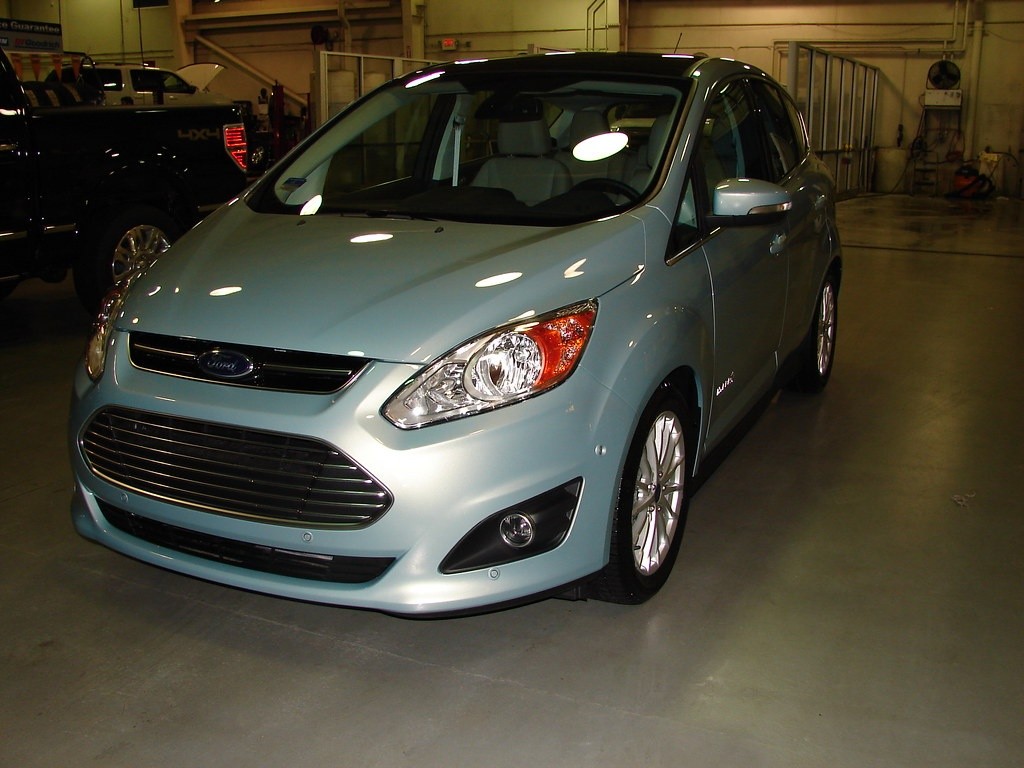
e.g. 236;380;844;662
472;105;728;216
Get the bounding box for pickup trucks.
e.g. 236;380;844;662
0;42;252;313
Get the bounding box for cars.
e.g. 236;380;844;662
64;49;847;621
44;62;234;110
